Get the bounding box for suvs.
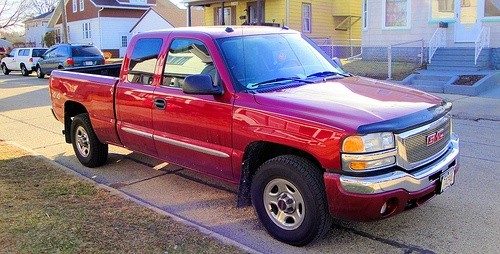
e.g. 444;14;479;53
0;48;48;76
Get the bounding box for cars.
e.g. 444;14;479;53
36;42;106;79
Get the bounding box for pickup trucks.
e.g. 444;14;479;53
48;26;459;247
